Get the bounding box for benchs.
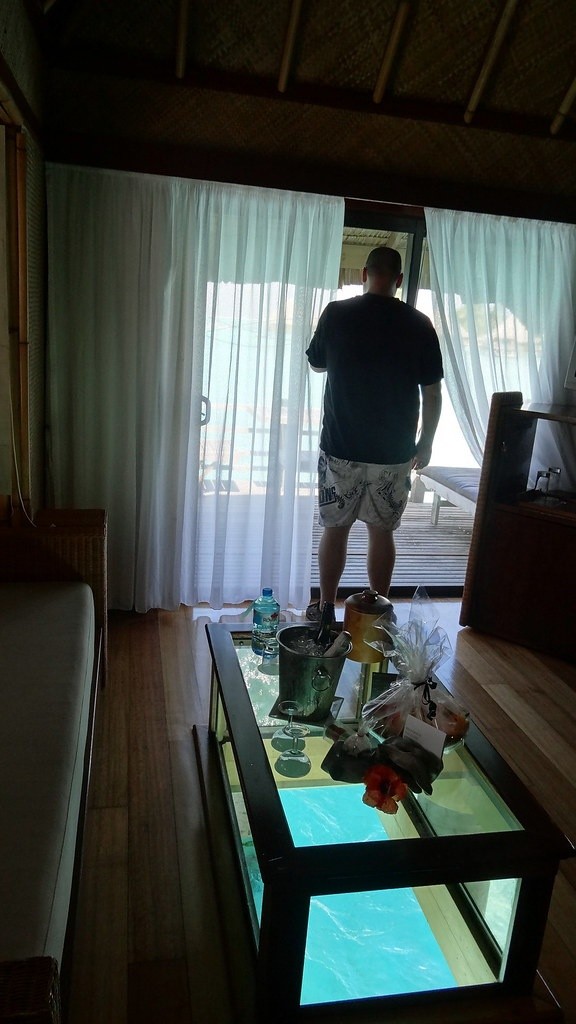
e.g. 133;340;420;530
0;507;109;1024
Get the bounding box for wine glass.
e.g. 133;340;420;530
271;701;305;752
274;723;312;777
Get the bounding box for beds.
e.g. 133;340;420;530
416;467;481;525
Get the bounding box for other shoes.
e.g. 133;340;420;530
305;600;336;622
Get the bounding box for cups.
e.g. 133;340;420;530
534;471;550;498
549;468;561;491
263;638;278;666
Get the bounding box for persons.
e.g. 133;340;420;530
305;246;444;621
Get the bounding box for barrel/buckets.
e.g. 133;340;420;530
276;625;353;721
343;590;393;664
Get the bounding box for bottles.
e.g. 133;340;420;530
316;601;336;645
326;631;351;658
252;588;280;655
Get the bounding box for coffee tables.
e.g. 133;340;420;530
194;621;576;1024
